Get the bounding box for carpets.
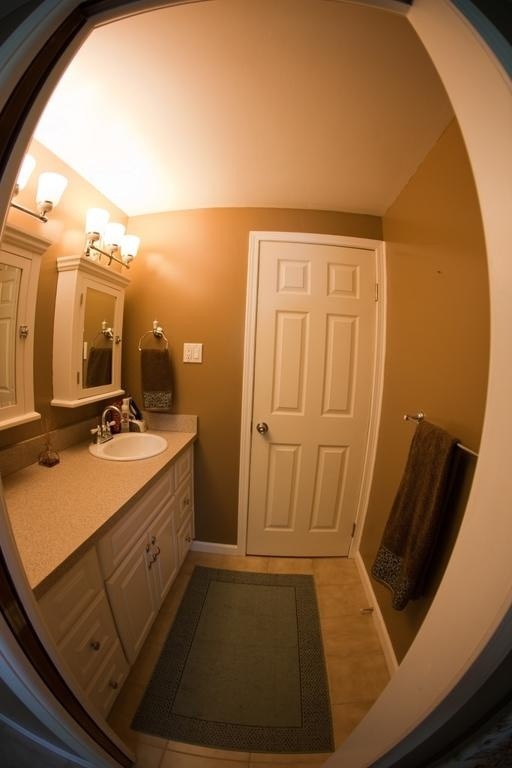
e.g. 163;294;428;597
129;564;335;754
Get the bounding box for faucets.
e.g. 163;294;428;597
101;405;126;440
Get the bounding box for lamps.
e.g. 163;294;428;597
84;207;140;273
10;154;67;222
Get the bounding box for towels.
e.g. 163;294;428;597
371;421;461;612
140;347;174;411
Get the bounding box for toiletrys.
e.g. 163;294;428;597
121;399;130;433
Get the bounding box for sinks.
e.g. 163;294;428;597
87;432;168;461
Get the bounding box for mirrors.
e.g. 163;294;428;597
51;257;130;408
1;222;53;431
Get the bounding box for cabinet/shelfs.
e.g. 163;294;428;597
97;468;178;667
38;446;128;725
173;446;194;567
